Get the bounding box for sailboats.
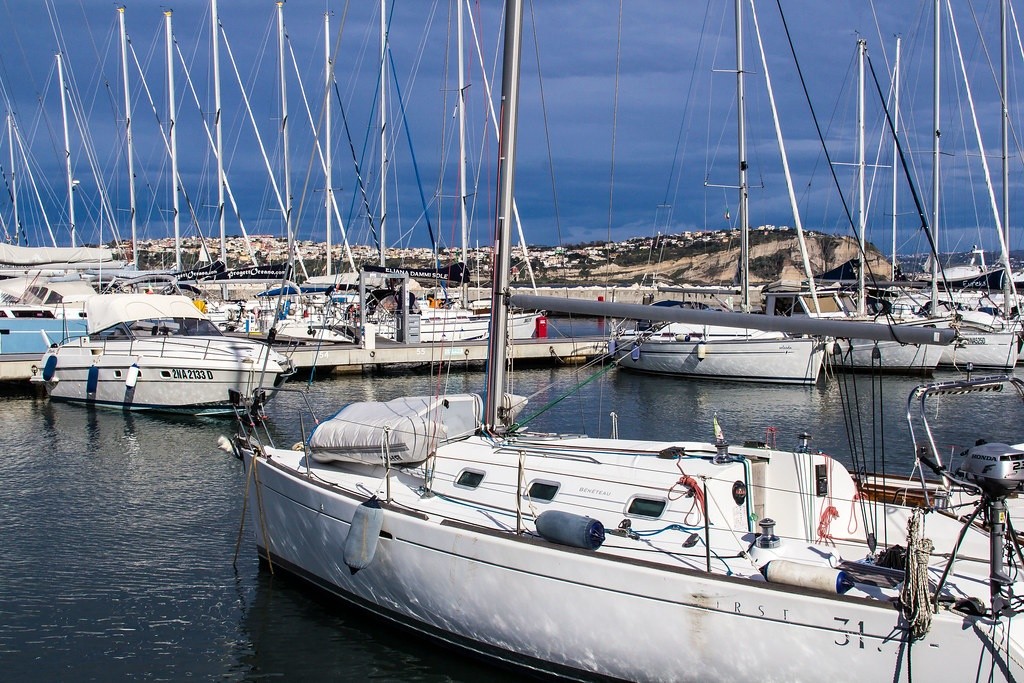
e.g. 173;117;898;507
0;0;1024;683
